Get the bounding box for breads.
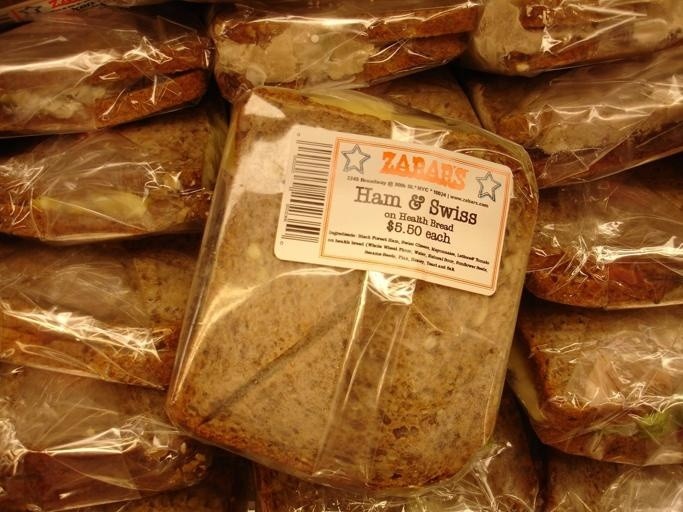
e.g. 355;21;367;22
377;65;484;129
461;72;537;133
0;361;213;512
204;0;479;105
465;0;683;78
523;173;683;310
0;245;199;391
499;44;682;190
543;455;683;512
507;305;683;467
1;16;213;132
56;461;237;511
235;384;547;511
165;88;539;490
1;103;229;246
